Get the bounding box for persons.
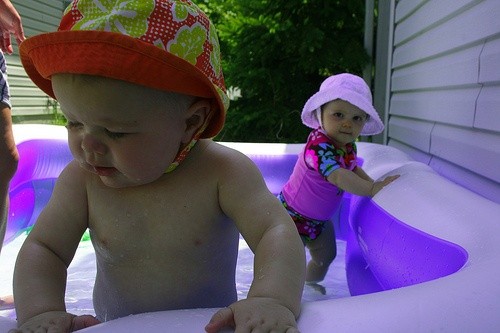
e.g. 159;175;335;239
7;0;305;333
0;0;26;310
276;73;400;295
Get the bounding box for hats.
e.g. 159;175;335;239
19;1;230;174
300;73;385;136
